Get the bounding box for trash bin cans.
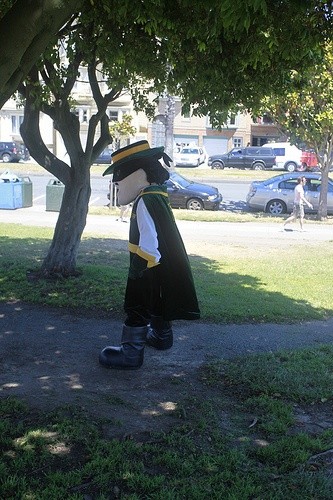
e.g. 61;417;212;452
18;176;32;208
0;178;23;210
46;179;65;211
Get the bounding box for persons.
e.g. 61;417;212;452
114;203;131;223
246;142;251;147
98;141;200;371
279;175;313;232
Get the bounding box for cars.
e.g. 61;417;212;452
107;169;223;210
247;172;333;214
176;142;206;167
91;148;114;164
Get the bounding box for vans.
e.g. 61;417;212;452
256;143;308;173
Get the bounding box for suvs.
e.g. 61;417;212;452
208;146;277;170
0;142;21;163
